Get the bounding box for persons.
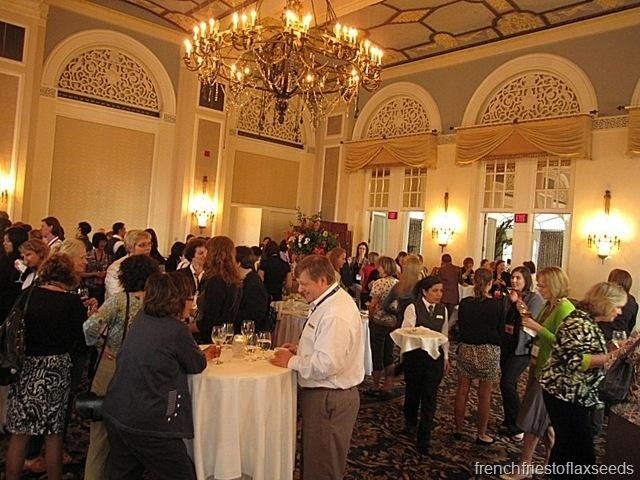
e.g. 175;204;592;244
0;210;640;480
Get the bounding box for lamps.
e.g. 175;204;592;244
183;0;384;131
432;192;456;253
587;190;622;264
189;176;214;229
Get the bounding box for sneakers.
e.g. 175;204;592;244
24;449;72;472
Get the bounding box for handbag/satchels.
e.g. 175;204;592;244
73;391;107;420
598;351;635;402
0;307;27;370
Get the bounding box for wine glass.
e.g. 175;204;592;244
611;328;628;349
14;259;26;284
100;261;108;271
517;300;535;325
78;287;89;301
210;321;272;365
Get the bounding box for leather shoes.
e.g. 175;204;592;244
454;431;462;440
476;422;525;444
499;470;532;479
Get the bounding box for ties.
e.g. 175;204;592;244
429;306;433;317
